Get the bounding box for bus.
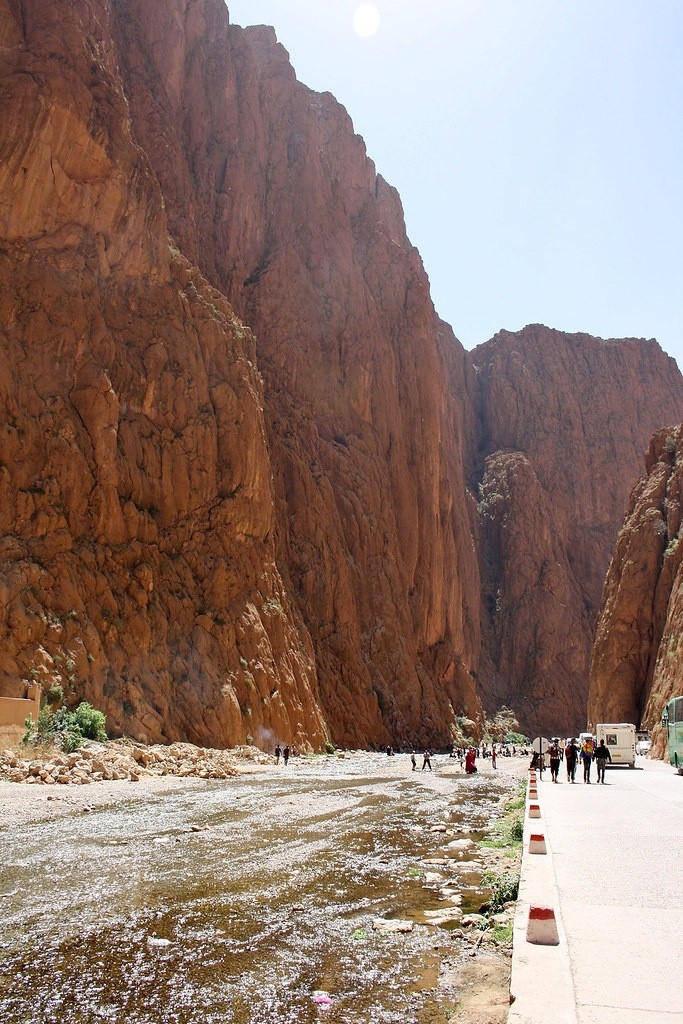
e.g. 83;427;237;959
661;696;683;775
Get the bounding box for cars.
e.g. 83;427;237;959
636;736;653;756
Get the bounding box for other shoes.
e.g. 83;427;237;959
567;775;575;784
583;780;592;784
597;779;605;784
552;778;558;784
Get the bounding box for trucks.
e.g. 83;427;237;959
596;723;635;768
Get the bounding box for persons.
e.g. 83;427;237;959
283;745;289;766
545;737;612;783
275;745;282;765
386;738;529;775
